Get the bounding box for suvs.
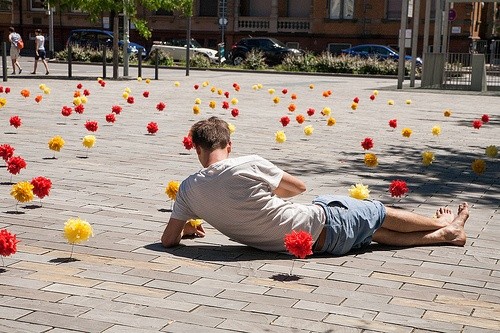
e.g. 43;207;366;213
66;29;148;62
228;34;303;67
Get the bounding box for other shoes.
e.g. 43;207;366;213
12;72;15;74
18;68;22;74
45;72;49;75
31;72;36;74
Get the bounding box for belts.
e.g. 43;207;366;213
312;204;328;257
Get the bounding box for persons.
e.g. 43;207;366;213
9;27;23;74
160;116;470;256
31;29;50;75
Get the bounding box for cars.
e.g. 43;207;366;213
340;44;424;72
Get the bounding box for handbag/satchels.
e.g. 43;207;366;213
17;39;23;49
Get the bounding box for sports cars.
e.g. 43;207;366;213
147;37;226;66
